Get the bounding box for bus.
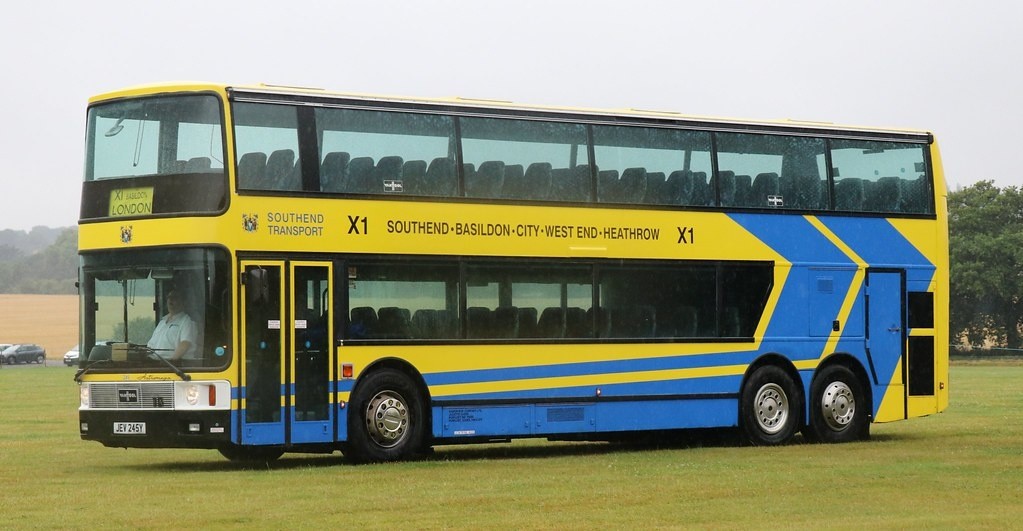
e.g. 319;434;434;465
72;79;950;463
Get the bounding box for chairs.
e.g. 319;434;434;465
350;305;743;339
162;158;212;173
239;149;927;213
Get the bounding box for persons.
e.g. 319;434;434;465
146;288;197;361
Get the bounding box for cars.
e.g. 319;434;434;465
0;344;47;365
0;344;15;354
63;340;118;367
